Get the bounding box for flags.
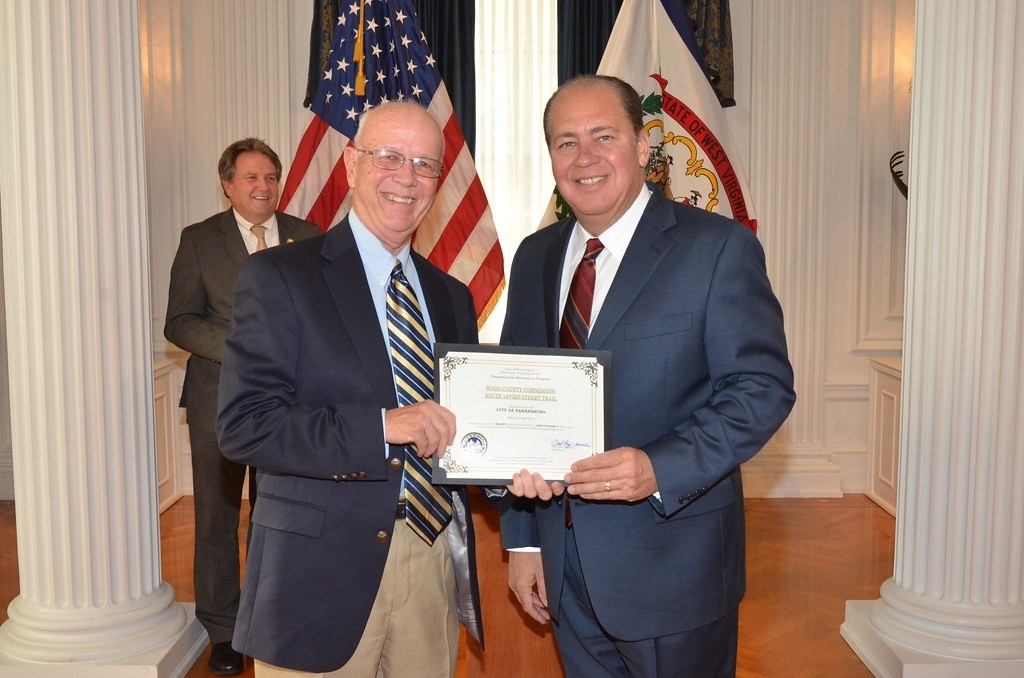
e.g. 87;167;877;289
538;0;757;236
278;0;507;332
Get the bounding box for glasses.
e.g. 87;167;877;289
349;145;445;179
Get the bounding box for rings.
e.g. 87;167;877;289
606;482;610;491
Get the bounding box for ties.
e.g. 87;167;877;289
249;224;268;250
386;259;454;548
559;238;605;529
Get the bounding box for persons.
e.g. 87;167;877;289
216;103;564;678
496;75;797;678
163;139;321;676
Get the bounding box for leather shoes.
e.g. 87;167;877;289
208;642;244;676
